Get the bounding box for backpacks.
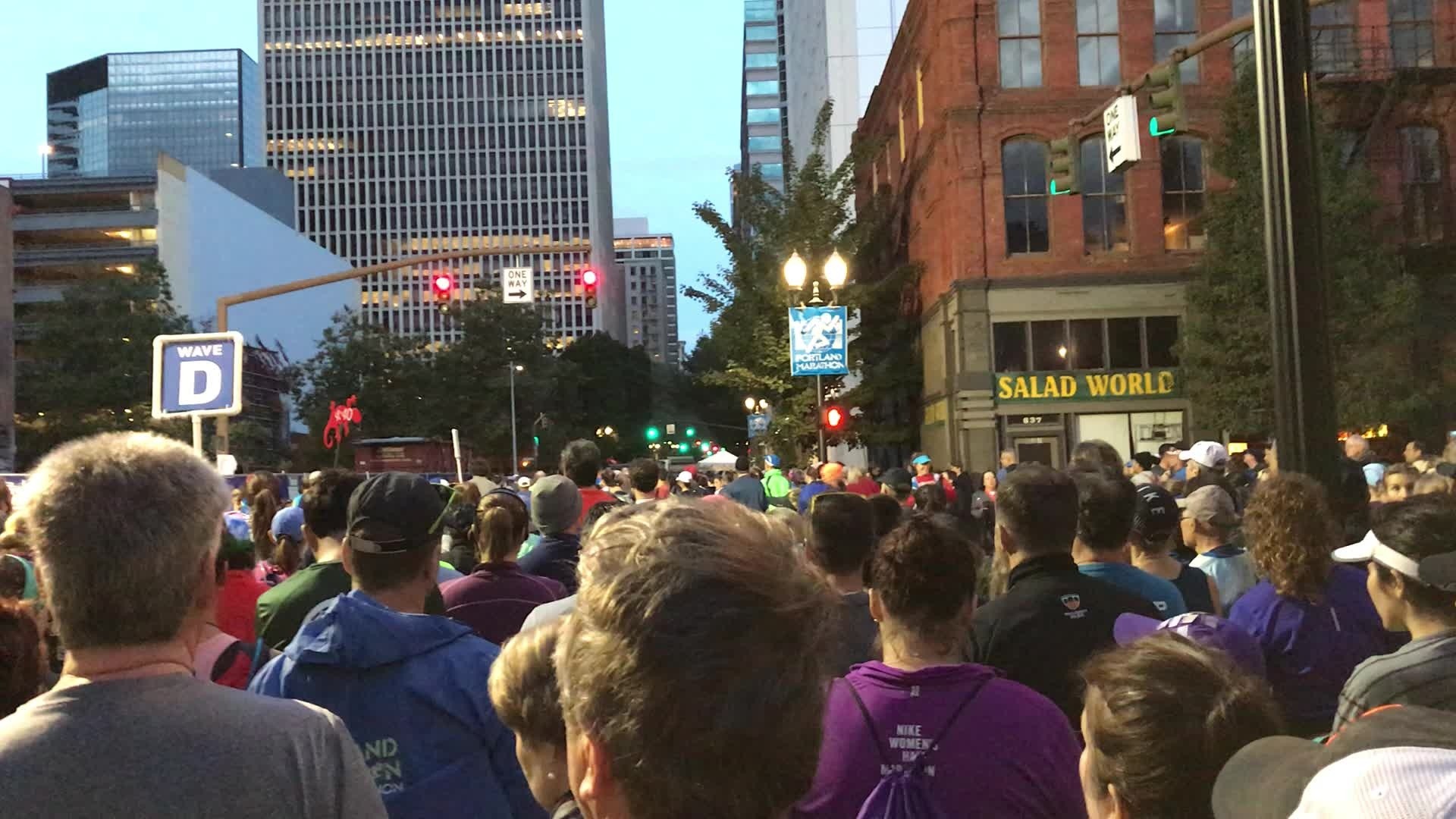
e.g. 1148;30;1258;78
836;674;986;819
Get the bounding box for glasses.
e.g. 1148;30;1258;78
428;482;462;534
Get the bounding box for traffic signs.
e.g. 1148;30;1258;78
503;266;534;303
1101;95;1141;174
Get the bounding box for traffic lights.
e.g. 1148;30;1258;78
645;429;661;440
583;269;598;308
1047;135;1081;197
685;422;695;438
437;274;453;316
1144;64;1189;137
823;406;843;428
533;435;541;446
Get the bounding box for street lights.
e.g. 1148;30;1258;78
782;245;849;465
744;397;769;472
509;360;525;475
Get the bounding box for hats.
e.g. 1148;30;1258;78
911;454;932;464
763;454;781;468
875;467;913;492
347;471;444;554
529;474;582;535
1178;440;1227;468
1175;485;1235;523
271;506;305;542
682;464;697;473
676;471;692;483
1158;440;1189;456
1212;705;1456;819
1436;461;1456;479
1114;612;1268;682
518;476;530;487
1132;483;1178;541
735;456;749;471
1331;529;1421;581
1363;463;1386;486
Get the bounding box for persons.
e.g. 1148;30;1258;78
1073;471;1189;621
1332;518;1455;737
257;469;353;651
1229;472;1413;740
0;430;1456;819
548;494;844;819
963;460;1166;739
788;515;1089;819
0;429;388;819
247;471;554;819
438;486;570;648
1132;483;1228;618
1176;484;1260;618
1079;634;1293;819
